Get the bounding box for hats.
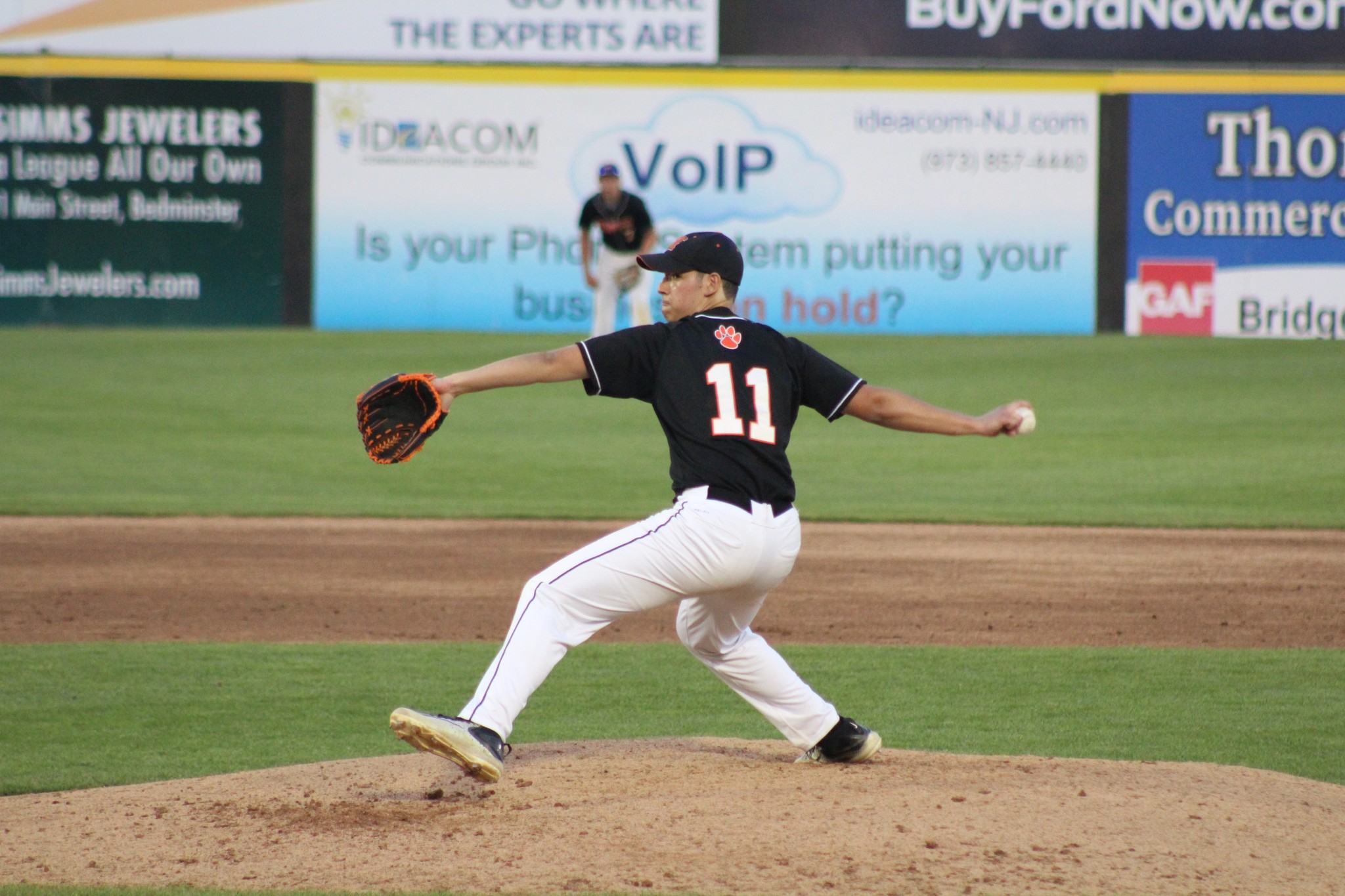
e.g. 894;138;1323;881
600;165;618;177
636;232;743;286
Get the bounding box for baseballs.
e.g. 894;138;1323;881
1009;406;1036;435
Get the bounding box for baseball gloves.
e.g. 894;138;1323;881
614;265;640;292
357;373;450;464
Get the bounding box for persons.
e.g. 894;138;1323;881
579;164;658;340
356;231;1030;786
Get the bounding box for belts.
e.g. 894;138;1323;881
706;487;793;517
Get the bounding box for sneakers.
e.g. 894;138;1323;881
389;708;511;783
793;715;883;764
